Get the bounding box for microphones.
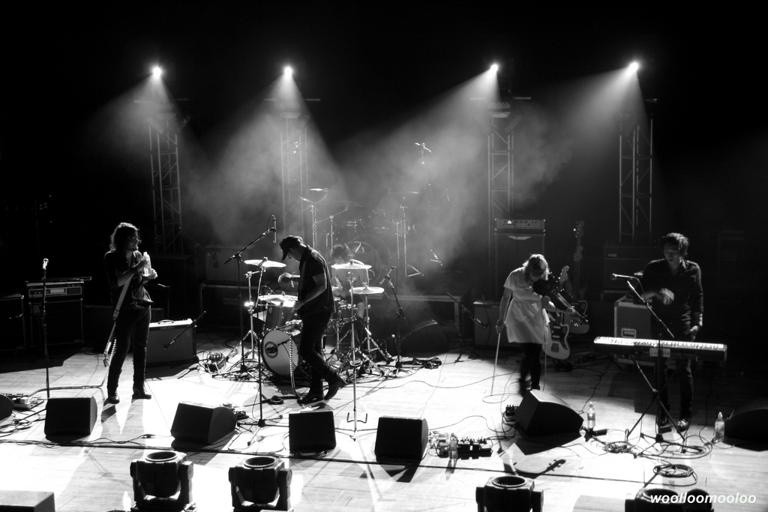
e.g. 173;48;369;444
257;257;268;268
609;273;637;282
42;258;49;270
272;217;277;248
379;267;396;286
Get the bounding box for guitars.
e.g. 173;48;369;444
543;265;570;360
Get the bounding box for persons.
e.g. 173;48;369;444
495;254;556;397
331;244;370;374
104;224;151;404
280;236;344;404
633;232;704;433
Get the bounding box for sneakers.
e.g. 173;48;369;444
131;391;151;400
106;388;120;404
656;411;670;424
676;416;692;430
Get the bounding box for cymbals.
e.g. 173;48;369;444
348;286;384;295
258;295;297;307
244;259;286;268
330;263;371;270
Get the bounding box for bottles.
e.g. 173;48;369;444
448;433;458;460
713;412;726;442
586;403;596;428
142;251;151;275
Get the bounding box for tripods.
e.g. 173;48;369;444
236;315;289;445
330;311;381;442
211;313;410;381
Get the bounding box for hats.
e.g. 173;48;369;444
280;235;299;260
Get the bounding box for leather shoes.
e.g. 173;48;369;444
297;389;324;403
323;377;347;400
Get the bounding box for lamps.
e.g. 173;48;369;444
476;475;545;512
625;486;690;512
228;454;294;511
129;451;194;511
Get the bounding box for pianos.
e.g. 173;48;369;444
593;336;727;363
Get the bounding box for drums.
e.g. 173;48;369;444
265;303;296;330
260;325;303;376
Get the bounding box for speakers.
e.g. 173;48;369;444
147;319;196;364
23;278;84;356
289;411;336;454
44;396;97;442
514;389;584;442
374;416;428;462
490;230;545;302
171;402;237;445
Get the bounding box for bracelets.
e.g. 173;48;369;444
497;320;504;325
302;299;307;306
695;323;702;329
131;265;138;274
